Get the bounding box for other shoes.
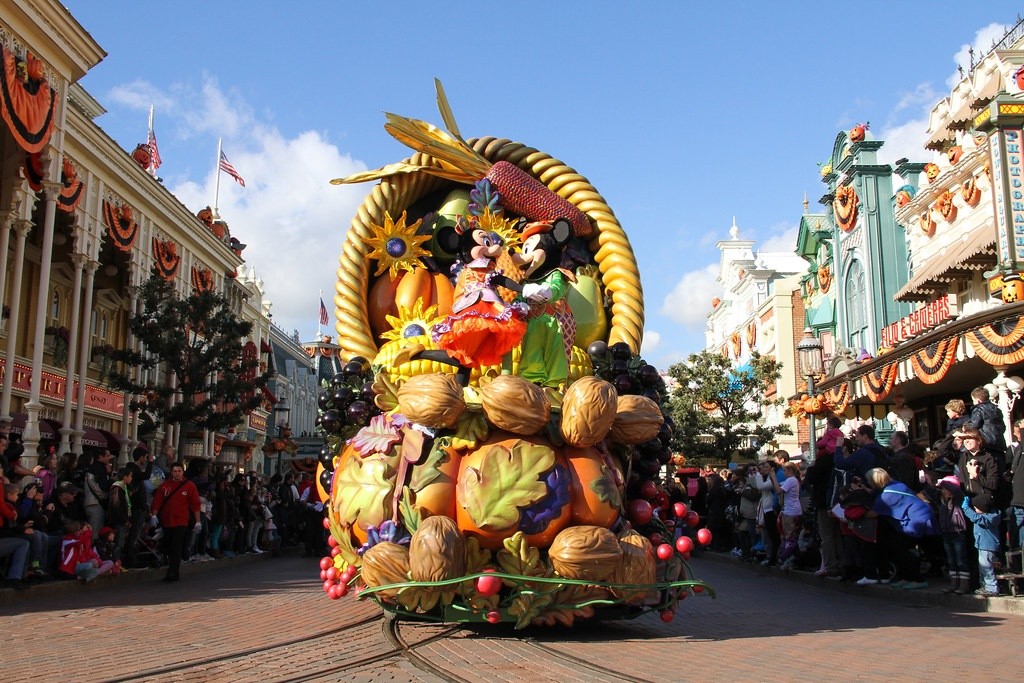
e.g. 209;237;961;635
0;545;272;590
974;588;999;597
890;579;910;589
903;581;929;590
880;579;890;584
707;542;828;577
856;577;878;585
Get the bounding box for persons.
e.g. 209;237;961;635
658;387;1024;598
0;421;323;591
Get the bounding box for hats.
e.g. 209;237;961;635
98;527;116;540
972;493;995;513
943;474;961;488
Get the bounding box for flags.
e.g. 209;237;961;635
321;299;328;326
149;126;162;168
220;152;245;187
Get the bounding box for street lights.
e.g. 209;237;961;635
272;394;291;491
793;325;827;466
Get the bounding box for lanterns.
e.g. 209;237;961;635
927;166;939;182
850;128;864;142
135;149;150;169
1002;277;1024;303
198;206;213;226
804;398;822;413
274;441;285;450
713;298;720;308
673;456;685;464
948;146;961;164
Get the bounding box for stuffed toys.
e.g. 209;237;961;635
507;218;574;389
392;227;544;396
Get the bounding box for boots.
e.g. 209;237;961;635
942;578;959;593
954;579;970;594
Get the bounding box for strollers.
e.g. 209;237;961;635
122;514;165;570
828;482;898;586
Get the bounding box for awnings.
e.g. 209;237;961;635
894;226;995;303
